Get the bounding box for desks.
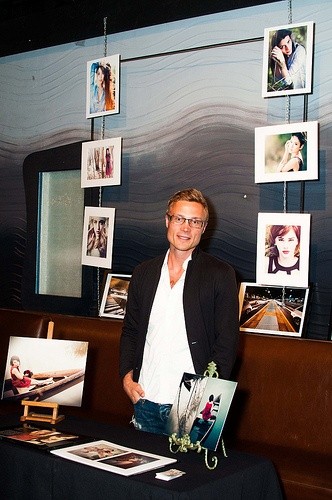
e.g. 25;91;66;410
0;414;288;500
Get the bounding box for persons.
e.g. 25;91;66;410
86;216;108;258
264;225;301;279
10;354;54;388
94;149;102;178
276;133;306;173
201;394;217;422
104;148;113;177
270;29;306;90
118;189;239;450
90;65;107;114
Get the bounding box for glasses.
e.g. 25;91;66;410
167;215;205;229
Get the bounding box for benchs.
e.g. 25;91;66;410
0;308;332;500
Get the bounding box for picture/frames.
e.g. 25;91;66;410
262;21;313;98
99;274;132;320
86;55;120;119
81;207;115;269
255;120;318;184
81;136;121;188
238;282;309;338
256;212;311;289
